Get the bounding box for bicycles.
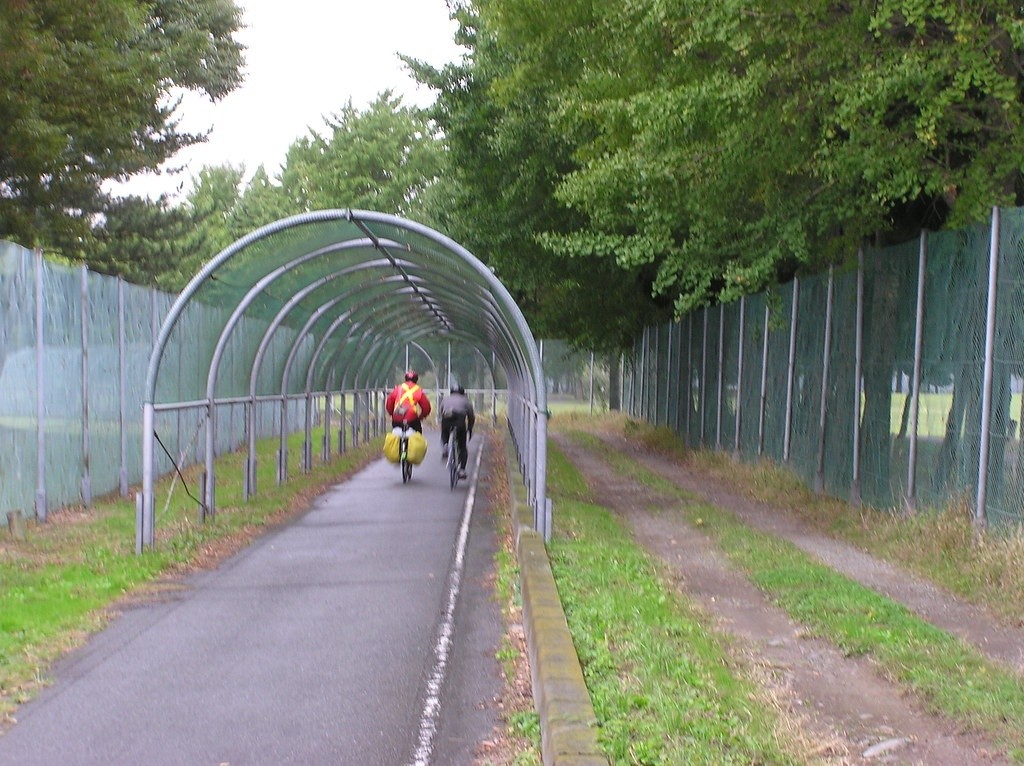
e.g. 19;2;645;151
401;421;412;484
444;423;472;491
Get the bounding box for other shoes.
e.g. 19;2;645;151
443;443;449;457
459;469;466;478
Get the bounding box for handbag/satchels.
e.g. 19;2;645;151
382;432;401;463
406;431;427;464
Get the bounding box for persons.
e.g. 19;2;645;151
385;370;431;435
437;383;476;479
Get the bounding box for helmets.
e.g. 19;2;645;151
451;384;464;394
405;371;419;383
